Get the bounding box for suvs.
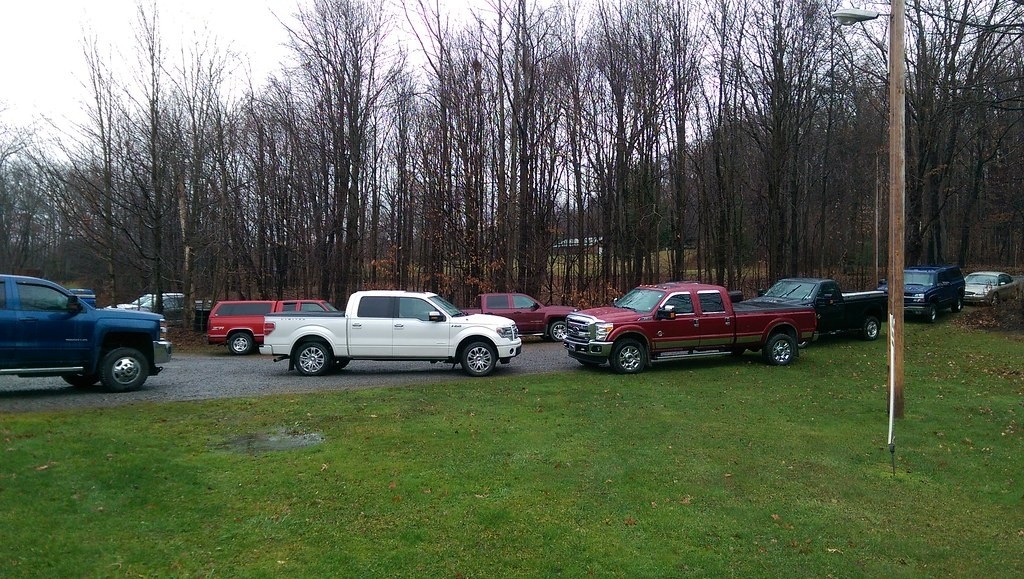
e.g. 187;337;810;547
58;288;96;310
1;273;173;392
877;264;966;324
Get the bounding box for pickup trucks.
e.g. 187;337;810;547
459;293;582;343
206;298;340;356
740;278;889;351
104;291;213;314
561;281;819;377
258;290;522;378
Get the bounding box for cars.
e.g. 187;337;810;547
961;271;1021;307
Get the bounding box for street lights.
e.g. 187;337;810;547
832;7;907;419
